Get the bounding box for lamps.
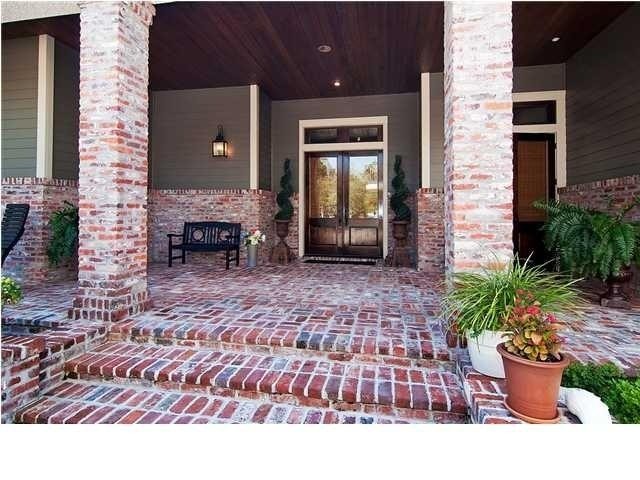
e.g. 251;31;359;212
210;125;228;157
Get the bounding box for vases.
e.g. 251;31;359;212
247;244;257;268
495;343;572;423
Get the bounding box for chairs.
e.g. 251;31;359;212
1;202;31;265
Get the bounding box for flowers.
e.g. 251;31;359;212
243;228;266;249
500;288;564;365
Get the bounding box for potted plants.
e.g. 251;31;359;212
270;157;295;264
389;154;413;266
434;248;585;378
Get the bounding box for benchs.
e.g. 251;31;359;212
167;221;239;271
